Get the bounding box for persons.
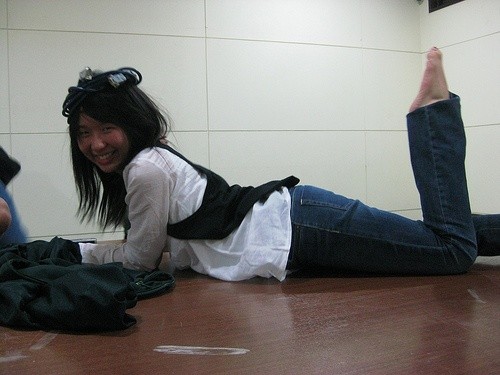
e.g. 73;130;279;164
0;145;28;248
62;46;500;283
